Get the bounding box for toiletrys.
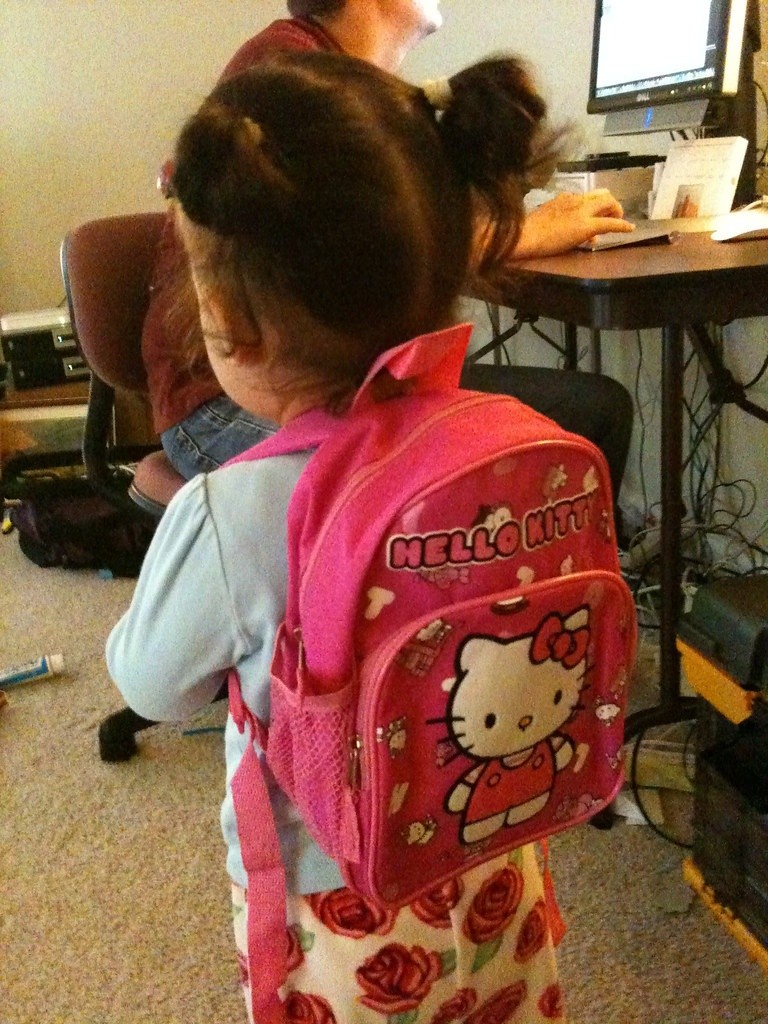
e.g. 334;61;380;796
0;653;66;691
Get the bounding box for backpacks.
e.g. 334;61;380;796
10;473;160;580
214;322;636;1024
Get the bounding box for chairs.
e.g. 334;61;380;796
62;215;230;763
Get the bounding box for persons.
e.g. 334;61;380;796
142;0;633;501
106;52;569;1024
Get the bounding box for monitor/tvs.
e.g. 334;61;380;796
586;0;762;212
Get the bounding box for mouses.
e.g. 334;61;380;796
711;210;768;242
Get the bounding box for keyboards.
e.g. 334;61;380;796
576;217;688;252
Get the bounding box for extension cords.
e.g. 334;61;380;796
617;529;663;572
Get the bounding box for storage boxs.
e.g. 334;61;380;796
675;577;768;968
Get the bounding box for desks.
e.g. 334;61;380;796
458;218;768;830
